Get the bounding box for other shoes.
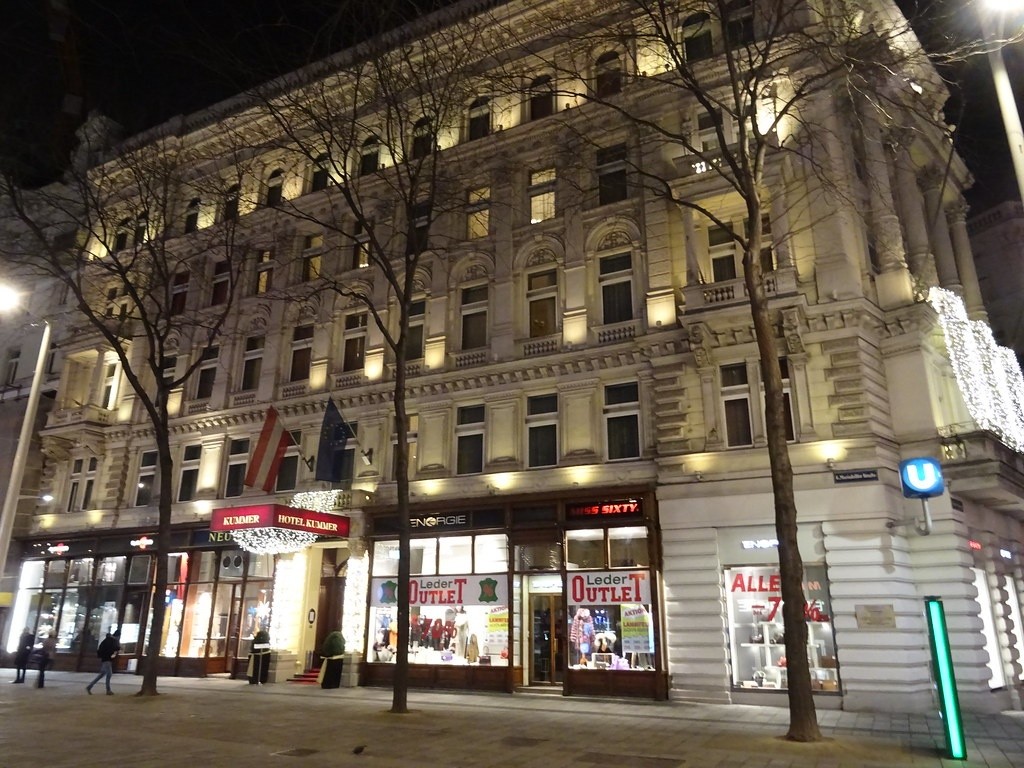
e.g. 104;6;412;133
86;687;92;694
106;691;114;695
14;678;24;683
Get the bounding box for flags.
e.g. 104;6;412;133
316;396;348;482
244;406;293;493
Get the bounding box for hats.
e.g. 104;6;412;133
113;630;120;636
49;629;57;637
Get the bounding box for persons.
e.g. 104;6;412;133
13;627;35;683
35;630;58;688
87;630;121;695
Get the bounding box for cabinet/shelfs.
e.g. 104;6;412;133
735;596;835;689
